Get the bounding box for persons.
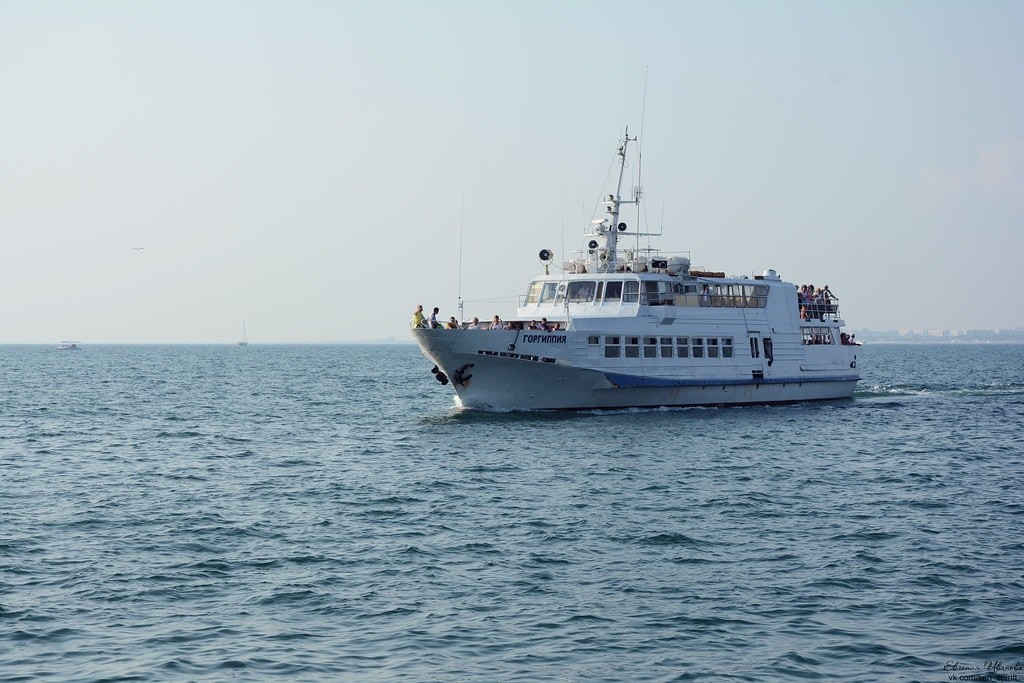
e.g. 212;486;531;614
699;283;735;307
445;317;459;329
468;317;480;329
795;284;839;322
528;320;538;330
552;323;560;332
802;332;855;345
537;318;550;330
427;307;442;329
481;315;517;330
413;305;426;329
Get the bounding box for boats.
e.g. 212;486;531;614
408;125;863;409
